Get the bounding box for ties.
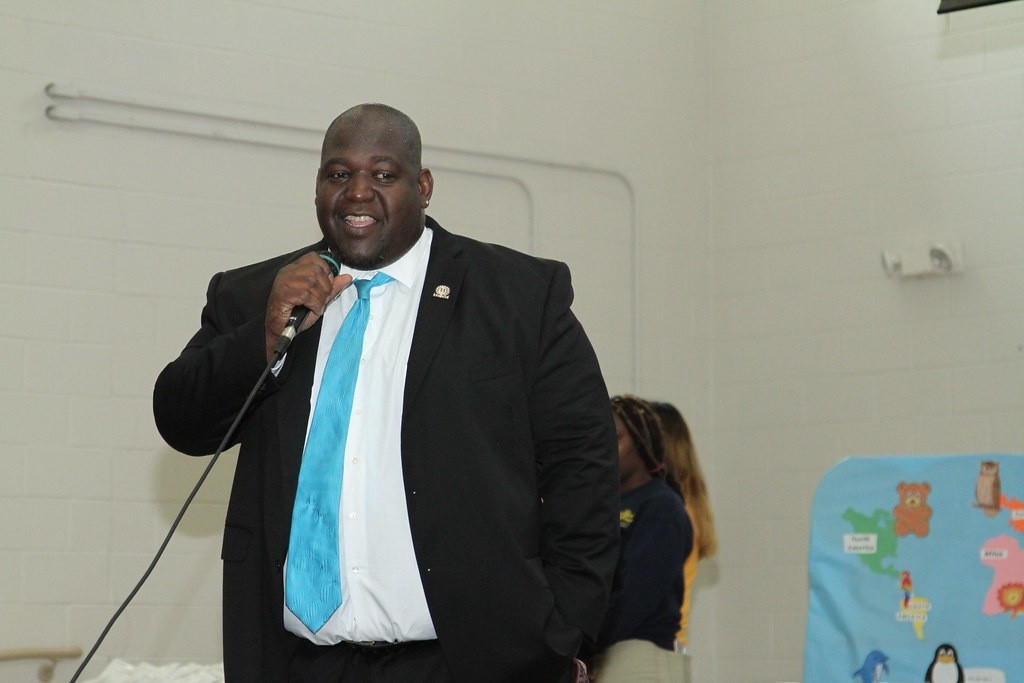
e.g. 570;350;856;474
285;271;397;632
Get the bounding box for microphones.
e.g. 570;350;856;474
274;253;340;360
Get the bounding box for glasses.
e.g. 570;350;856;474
611;397;645;416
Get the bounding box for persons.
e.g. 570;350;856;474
643;399;717;656
151;103;624;683
583;395;694;681
